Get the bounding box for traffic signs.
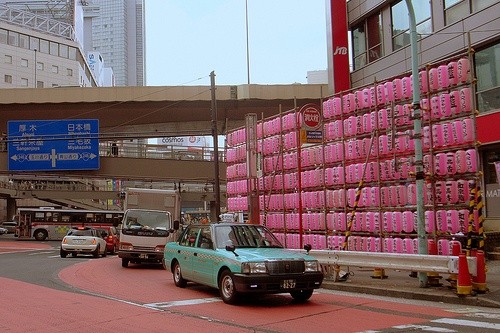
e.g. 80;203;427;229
6;118;101;172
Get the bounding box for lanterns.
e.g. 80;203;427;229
225;56;480;255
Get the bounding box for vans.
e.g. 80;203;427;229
1;221;17;234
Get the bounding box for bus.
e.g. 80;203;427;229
12;206;125;242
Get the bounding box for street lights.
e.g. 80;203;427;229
33;48;37;88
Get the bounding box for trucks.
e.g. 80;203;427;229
110;186;183;269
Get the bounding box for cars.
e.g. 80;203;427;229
0;227;8;234
59;225;108;258
161;210;326;306
89;225;120;256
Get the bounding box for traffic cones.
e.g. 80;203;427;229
425;240;441;278
369;267;389;279
474;252;489;292
456;253;474;296
446;242;463;281
